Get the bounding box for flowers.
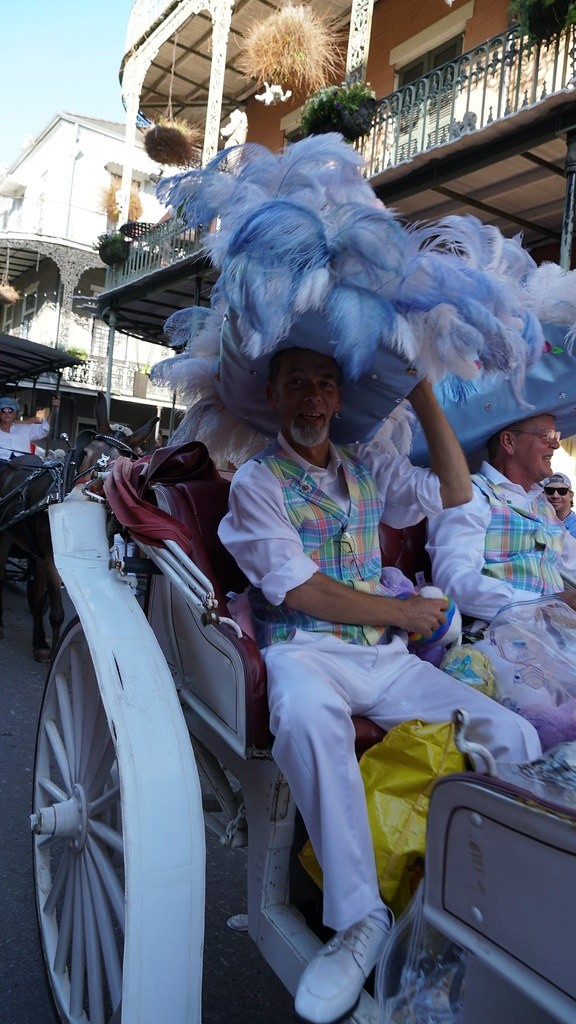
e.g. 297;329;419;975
92;233;125;250
301;81;377;136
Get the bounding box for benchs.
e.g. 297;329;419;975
422;747;576;1024
141;483;449;911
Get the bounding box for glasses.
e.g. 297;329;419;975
0;407;13;413
507;429;561;442
544;487;569;495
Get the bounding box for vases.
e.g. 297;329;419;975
318;100;374;140
99;240;129;266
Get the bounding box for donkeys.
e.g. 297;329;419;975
0;391;160;664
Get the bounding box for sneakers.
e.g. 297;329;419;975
294;907;397;1024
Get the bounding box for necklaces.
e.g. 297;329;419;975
561;510;573;523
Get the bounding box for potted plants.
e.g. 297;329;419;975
506;0;576;46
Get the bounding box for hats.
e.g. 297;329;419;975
0;397;17;411
543;472;571;489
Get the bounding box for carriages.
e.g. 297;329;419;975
1;329;162;664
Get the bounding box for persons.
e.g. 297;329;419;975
543;472;576;540
218;347;540;1024
0;398;60;461
425;412;576;622
